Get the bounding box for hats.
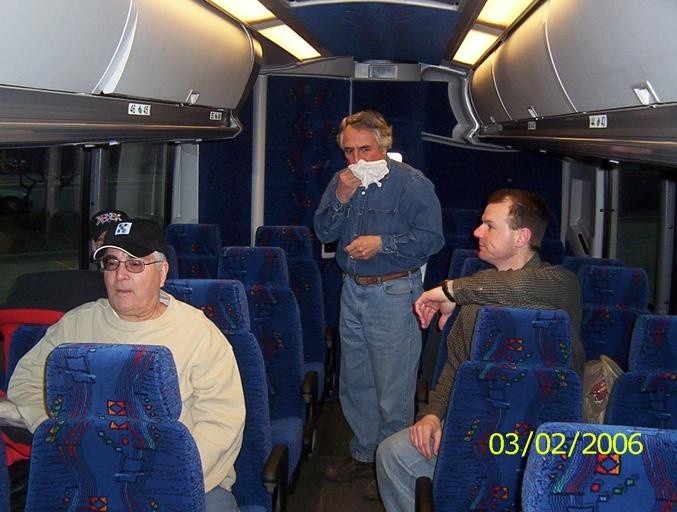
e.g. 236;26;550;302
91;217;167;262
90;209;130;241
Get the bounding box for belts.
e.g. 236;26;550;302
351;267;419;286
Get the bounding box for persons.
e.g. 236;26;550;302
371;187;584;512
0;194;57;304
87;208;132;270
5;217;247;511
312;108;447;485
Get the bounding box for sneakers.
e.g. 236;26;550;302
361;478;380;501
324;456;376;483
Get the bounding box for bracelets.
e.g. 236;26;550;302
439;278;455;305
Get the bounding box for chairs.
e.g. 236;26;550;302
1;223;335;508
417;229;674;507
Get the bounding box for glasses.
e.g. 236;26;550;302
97;255;164;273
